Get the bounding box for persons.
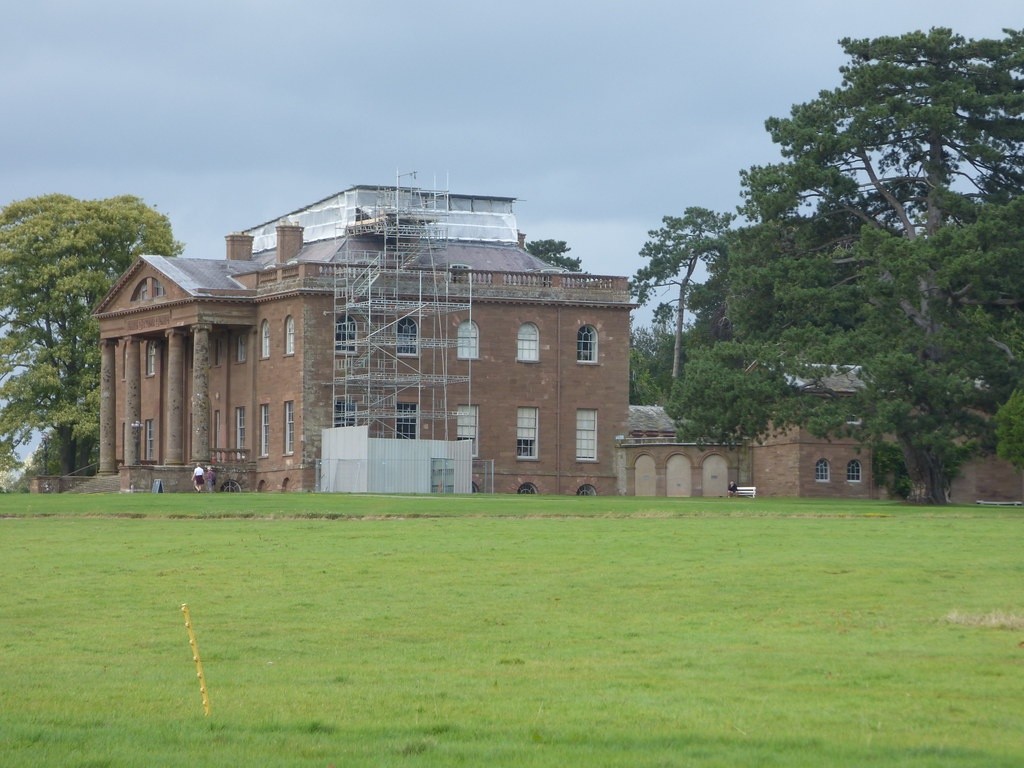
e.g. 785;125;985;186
205;467;215;493
728;481;738;498
192;463;205;492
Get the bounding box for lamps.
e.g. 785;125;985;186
41;433;54;442
130;421;144;430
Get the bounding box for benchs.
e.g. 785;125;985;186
727;486;756;497
977;501;1021;507
